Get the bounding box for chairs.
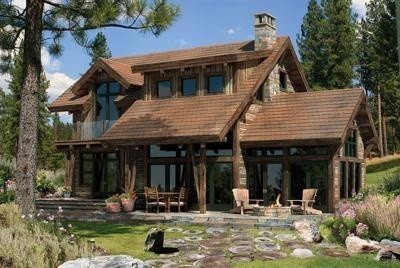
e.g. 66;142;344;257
170;187;188;212
232;189;264;215
144;186;165;213
285;189;318;215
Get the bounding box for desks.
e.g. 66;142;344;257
253;206;292;217
153;192;180;213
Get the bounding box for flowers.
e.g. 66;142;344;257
121;191;137;200
106;193;121;203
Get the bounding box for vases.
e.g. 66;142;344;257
105;202;122;213
121;198;136;211
